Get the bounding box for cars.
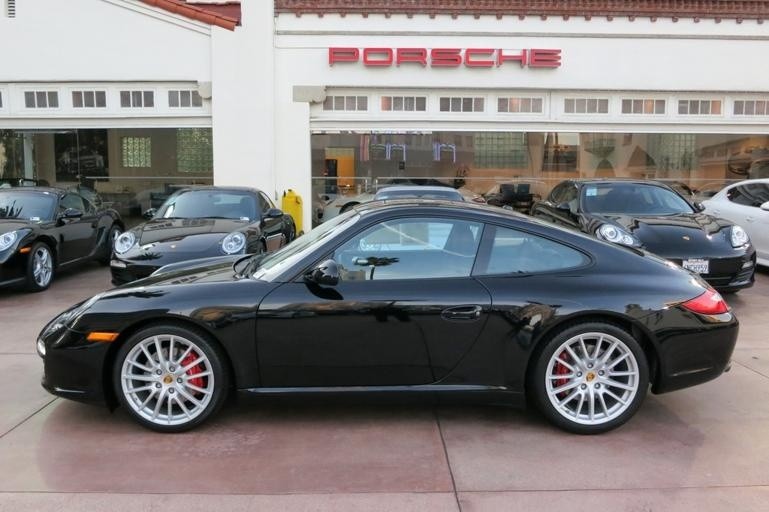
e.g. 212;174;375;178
0;185;127;292
109;186;297;287
529;177;756;293
700;178;769;267
35;197;740;434
357;184;479;251
